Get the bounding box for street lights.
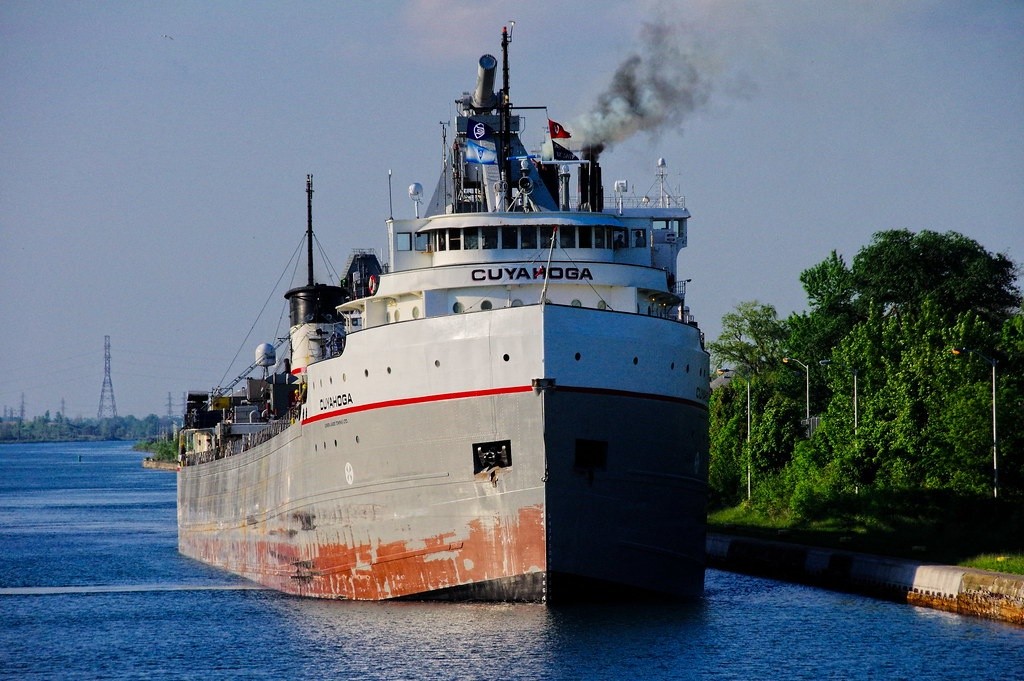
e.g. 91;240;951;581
819;360;859;496
781;357;810;439
953;345;997;498
717;368;750;506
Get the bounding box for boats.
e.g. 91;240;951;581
176;22;711;607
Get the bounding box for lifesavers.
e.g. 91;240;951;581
368;275;376;294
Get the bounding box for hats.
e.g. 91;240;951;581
294;389;298;393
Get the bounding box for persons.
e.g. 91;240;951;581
614;234;626;248
294;388;302;402
635;231;645;248
259;401;275;422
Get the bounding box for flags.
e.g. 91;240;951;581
549;119;572;139
552;140;579;161
465;118;499;165
289;368;303;384
